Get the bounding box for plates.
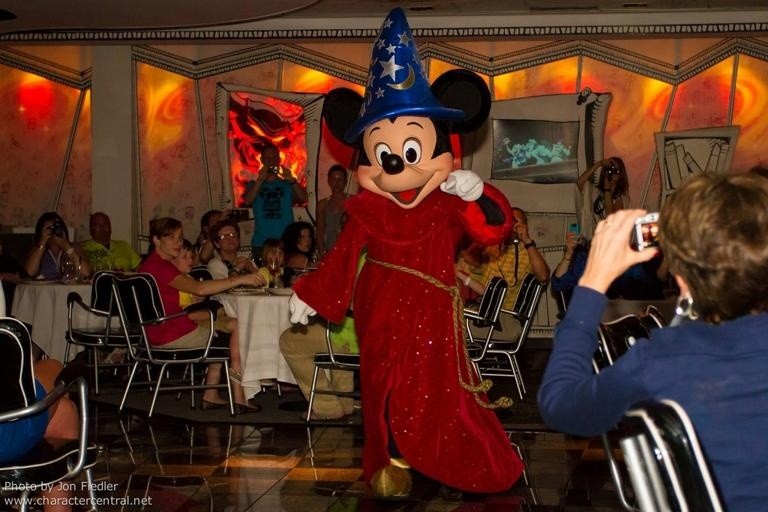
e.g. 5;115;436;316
226;288;259;296
21;278;59;284
269;288;293;295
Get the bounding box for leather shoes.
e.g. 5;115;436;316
232;402;263;415
200;400;230;410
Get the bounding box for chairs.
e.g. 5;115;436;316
306;323;376;422
0;316;99;512
101;269;239;417
603;399;725;511
474;272;544;401
65;269;135;394
461;277;509;384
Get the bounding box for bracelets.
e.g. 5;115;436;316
34;243;45;250
233;268;240;275
564;256;572;262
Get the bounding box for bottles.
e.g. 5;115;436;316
268;256;282;290
61;249;81;284
95;256;102;271
112;265;124;273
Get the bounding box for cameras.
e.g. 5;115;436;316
636;212;660;251
269;166;279;174
605;165;621;180
47;218;65;237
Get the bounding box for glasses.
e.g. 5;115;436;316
216;232;238;240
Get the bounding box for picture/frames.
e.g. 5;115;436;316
655;125;738;199
215;82;331;226
472;86;611;219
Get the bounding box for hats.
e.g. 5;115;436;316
342;7;466;144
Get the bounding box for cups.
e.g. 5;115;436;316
61;255;82;285
111;265;124;279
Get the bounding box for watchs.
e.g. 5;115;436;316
524;240;536;250
289;177;297;186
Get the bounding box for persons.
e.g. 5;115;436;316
194;208;232;266
207;220;259;281
315;165;357;260
251;239;283;288
576;157;632;252
23;212;92;281
82;212;145;277
463;206;551;341
538;171;768;512
0;359;79;512
282;222;318;289
245;144;308;270
551;231;667;300
137;218;267;413
288;8;527;498
172;238;243;384
